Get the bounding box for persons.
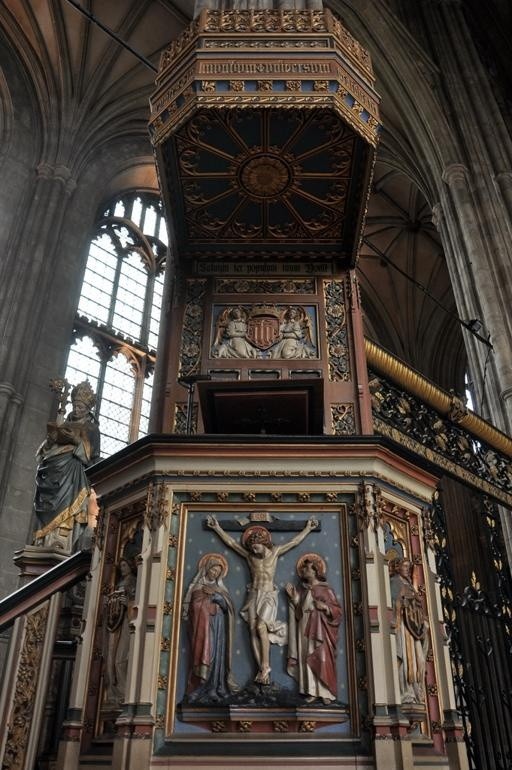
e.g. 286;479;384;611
34;382;100;553
207;512;319;687
212;308;258;359
390;558;430;740
188;556;236;704
284;560;344;706
267;304;318;359
108;555;136;706
